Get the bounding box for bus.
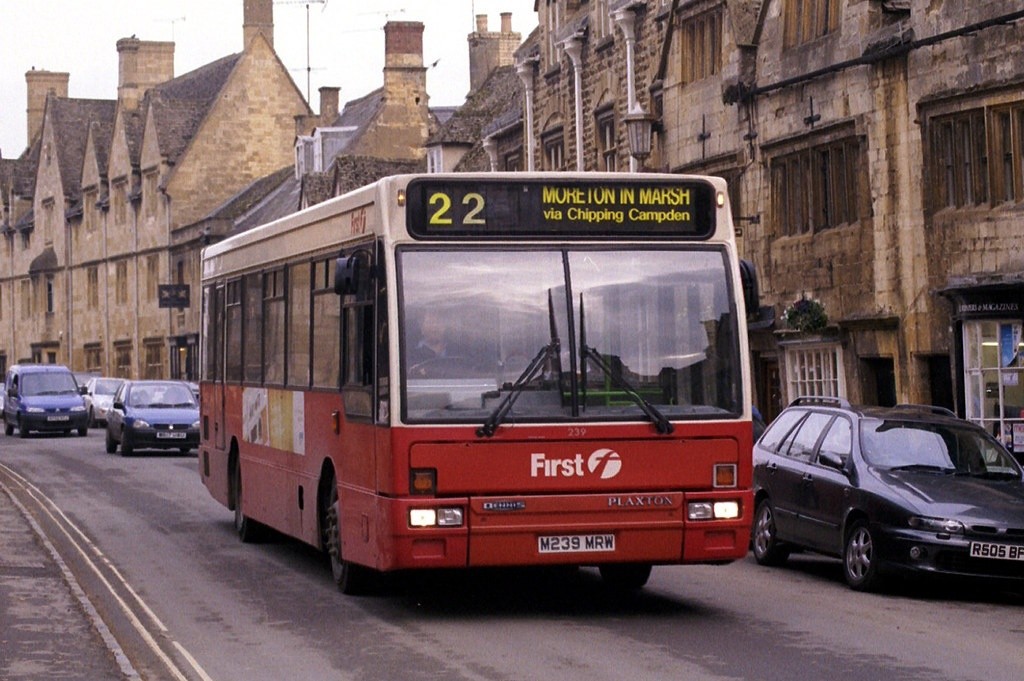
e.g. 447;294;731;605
197;166;755;603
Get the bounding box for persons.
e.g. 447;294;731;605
411;308;468;379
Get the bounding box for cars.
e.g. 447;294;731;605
105;380;199;457
752;385;1023;598
85;375;126;429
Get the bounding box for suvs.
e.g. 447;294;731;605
4;364;88;439
